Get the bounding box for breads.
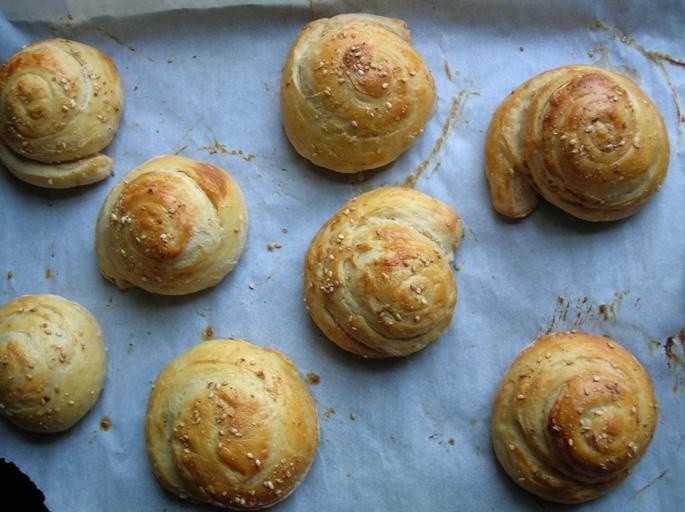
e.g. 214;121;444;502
0;294;106;435
304;186;466;360
0;37;123;190
145;339;320;512
491;329;658;505
280;13;437;174
95;154;249;297
484;63;670;223
